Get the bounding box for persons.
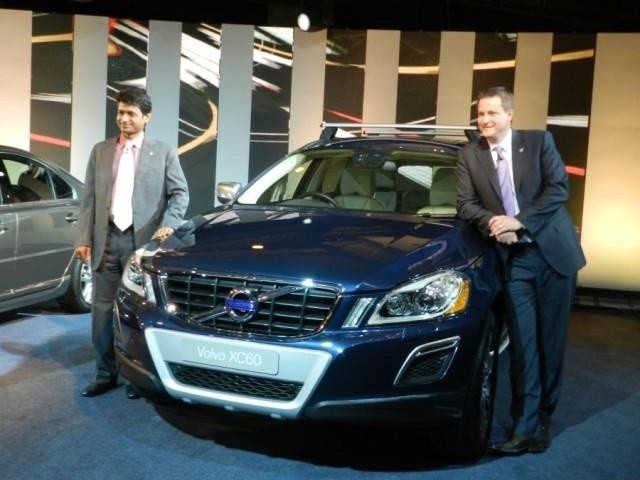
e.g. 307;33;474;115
75;86;189;400
451;85;588;456
138;219;196;260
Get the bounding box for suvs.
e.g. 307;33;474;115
111;117;511;466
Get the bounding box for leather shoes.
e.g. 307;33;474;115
81;382;140;398
488;425;550;453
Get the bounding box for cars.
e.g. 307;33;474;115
0;145;95;312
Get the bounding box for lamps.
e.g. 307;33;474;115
298;10;333;33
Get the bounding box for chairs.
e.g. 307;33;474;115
330;158;399;212
418;168;460;214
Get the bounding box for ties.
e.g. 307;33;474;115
113;142;135;232
493;146;515;219
